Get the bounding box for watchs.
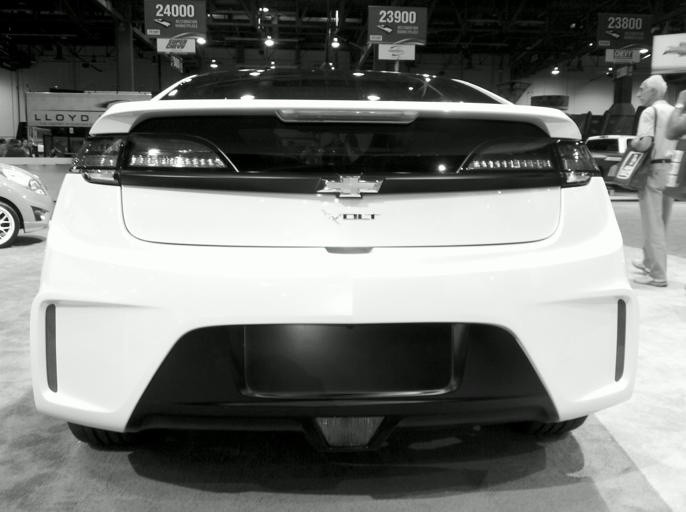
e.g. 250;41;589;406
675;102;684;109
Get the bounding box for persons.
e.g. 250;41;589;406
631;75;679;287
0;138;39;157
666;90;686;139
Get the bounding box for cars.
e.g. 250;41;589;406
26;63;640;460
0;162;53;249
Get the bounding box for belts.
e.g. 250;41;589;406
651;159;672;163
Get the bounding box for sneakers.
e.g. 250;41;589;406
631;259;668;286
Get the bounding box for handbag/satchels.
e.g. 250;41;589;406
614;143;653;190
